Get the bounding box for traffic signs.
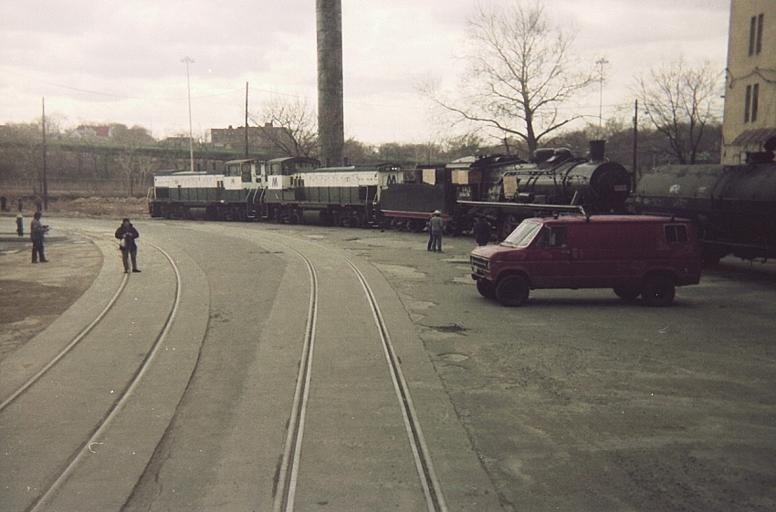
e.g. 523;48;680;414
180;57;196;171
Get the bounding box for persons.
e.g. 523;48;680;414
31;212;51;264
427;212;433;251
113;218;141;273
429;210;445;251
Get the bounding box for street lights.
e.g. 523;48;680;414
596;57;609;126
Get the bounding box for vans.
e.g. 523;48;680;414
469;216;705;307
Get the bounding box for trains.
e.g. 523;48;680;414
146;139;776;263
